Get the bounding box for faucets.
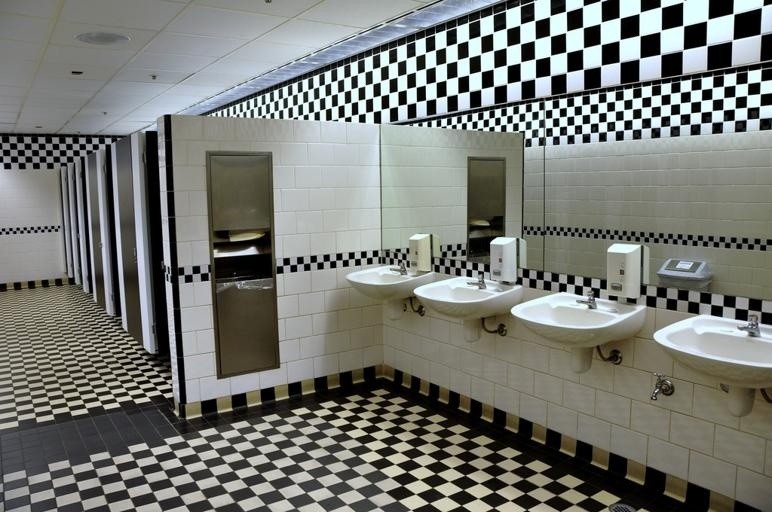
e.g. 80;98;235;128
737;315;761;337
466;275;486;289
576;292;597;309
390;264;408;275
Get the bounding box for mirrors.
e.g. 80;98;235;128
545;60;770;329
379;94;547;292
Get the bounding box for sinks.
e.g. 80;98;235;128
345;265;434;300
511;292;647;347
413;277;522;319
653;314;772;388
